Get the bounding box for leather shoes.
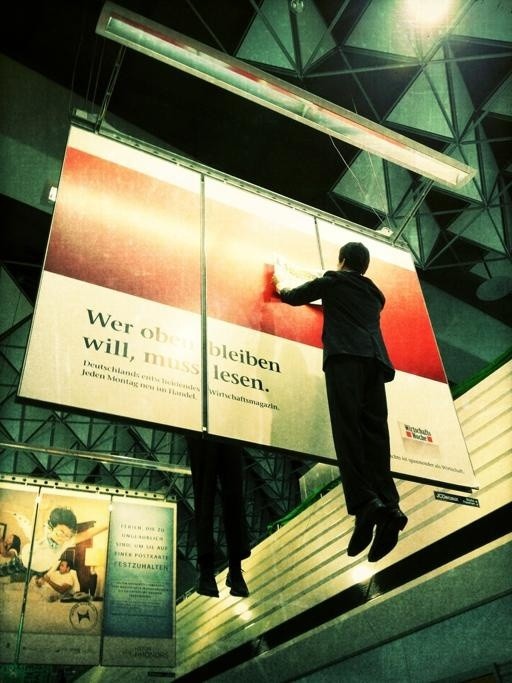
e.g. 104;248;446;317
198;573;219;598
226;568;249;597
368;509;407;562
348;497;388;556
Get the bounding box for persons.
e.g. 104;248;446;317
183;430;256;600
1;502;111;585
23;552;75;603
271;237;406;571
0;533;22;565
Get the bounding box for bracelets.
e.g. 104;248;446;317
48;578;51;583
